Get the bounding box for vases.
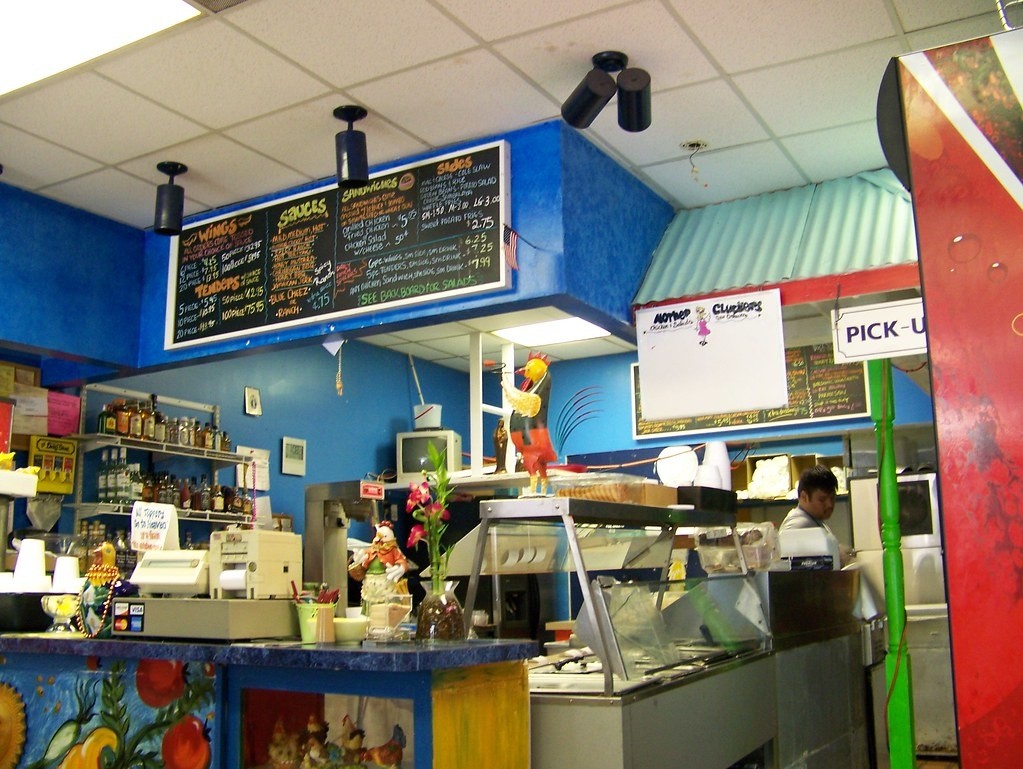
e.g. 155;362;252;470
414;580;467;647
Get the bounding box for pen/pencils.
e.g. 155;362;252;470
291;577;340;606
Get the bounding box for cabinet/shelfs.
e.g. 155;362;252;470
62;382;266;536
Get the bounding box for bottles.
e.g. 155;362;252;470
97;447;252;513
79;520;210;577
98;394;231;451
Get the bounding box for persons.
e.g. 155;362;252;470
493;418;508;469
778;464;851;566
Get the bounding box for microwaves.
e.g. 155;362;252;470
850;473;948;551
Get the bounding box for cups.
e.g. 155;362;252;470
41;596;82;633
415;404;443;427
297;604;336;645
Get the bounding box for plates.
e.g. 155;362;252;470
656;445;698;488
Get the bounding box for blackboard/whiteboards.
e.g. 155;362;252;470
164;137;510;350
630;340;872;440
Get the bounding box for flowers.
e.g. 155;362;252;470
405;439;460;594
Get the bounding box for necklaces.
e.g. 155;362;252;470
75;564;120;638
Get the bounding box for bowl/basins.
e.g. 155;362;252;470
735;544;772;569
694;546;736;572
27;534;81;557
333;617;369;645
694;441;731;490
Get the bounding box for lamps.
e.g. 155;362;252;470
153;161;190;237
561;51;653;133
332;104;371;190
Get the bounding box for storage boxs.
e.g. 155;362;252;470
678;486;738;514
816;453;850;495
0;470;38;498
790;453;819;490
625;483;677;507
746;452;793;492
